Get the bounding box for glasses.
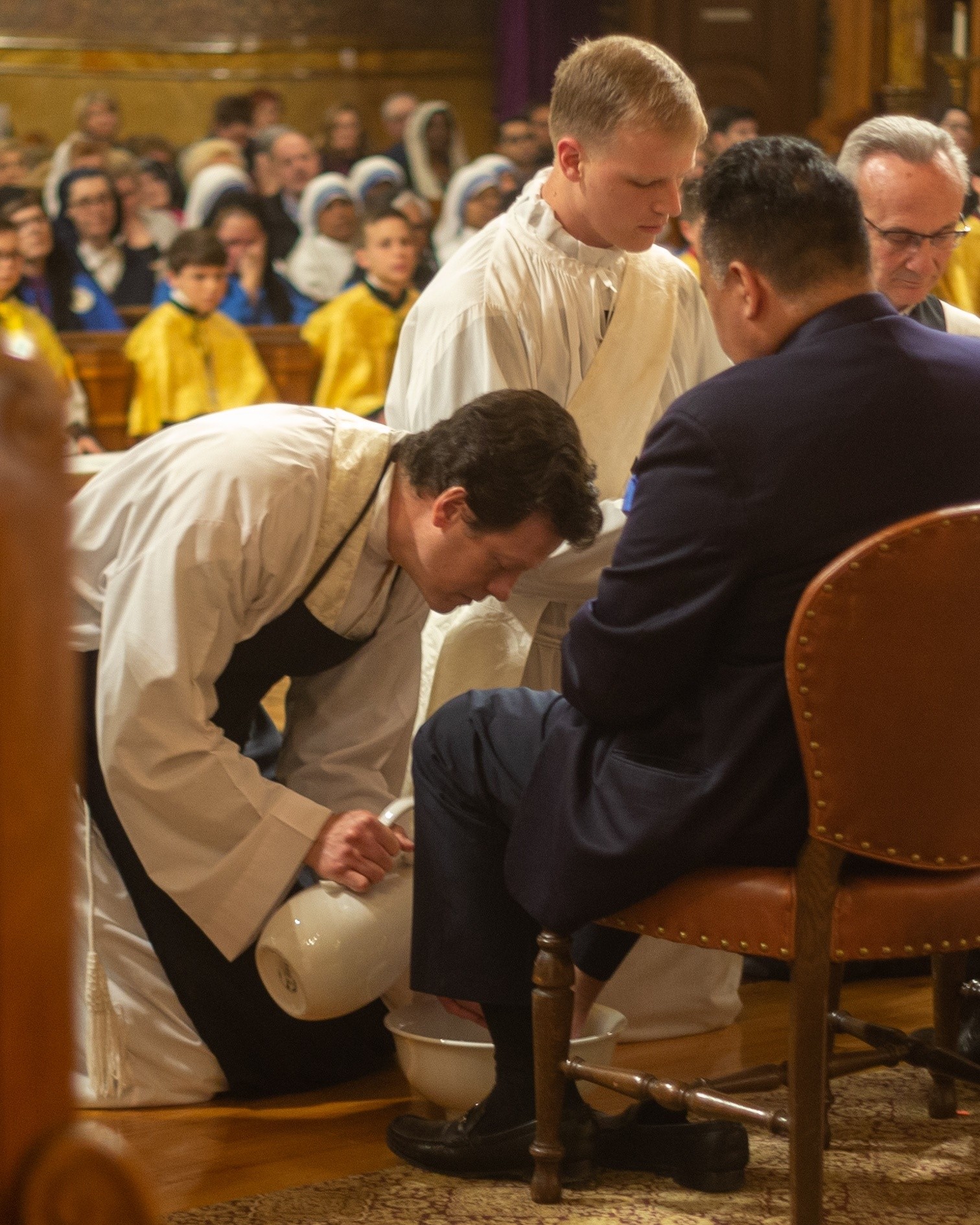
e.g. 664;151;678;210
864;212;971;253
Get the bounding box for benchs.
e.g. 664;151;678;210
58;304;327;453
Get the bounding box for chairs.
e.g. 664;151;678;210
531;504;980;1225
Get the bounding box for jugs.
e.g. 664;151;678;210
255;796;412;1019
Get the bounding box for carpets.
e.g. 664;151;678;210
160;1059;980;1225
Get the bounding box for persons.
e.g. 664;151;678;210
1;89;978;457
59;387;605;1109
384;135;979;1201
382;31;742;1043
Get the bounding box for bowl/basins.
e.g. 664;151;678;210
384;1001;627;1116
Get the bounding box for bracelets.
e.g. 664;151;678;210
66;422;87;441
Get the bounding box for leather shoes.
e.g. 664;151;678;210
599;1101;751;1195
385;1100;603;1189
